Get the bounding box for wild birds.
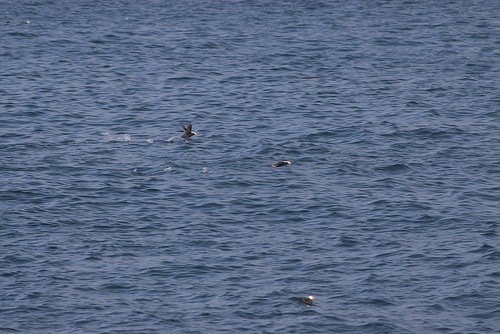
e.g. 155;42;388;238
179;123;197;143
287;295;317;305
272;160;292;167
